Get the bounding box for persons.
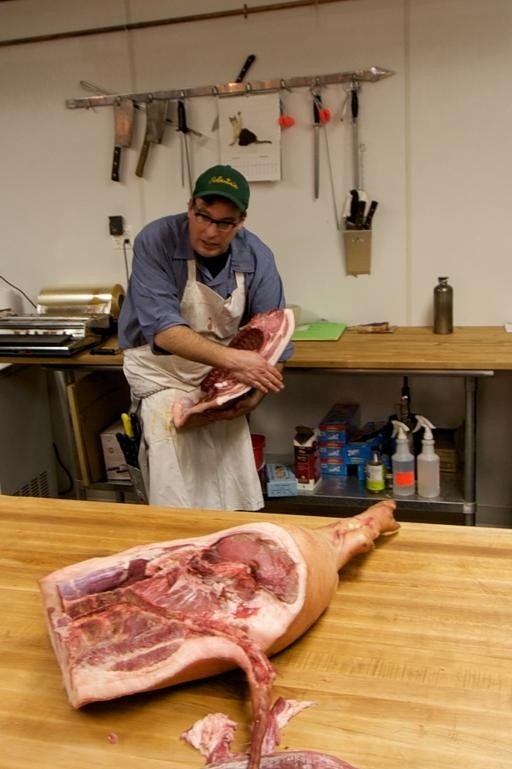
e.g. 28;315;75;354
115;164;296;512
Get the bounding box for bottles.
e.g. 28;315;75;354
432;276;455;335
365;449;386;495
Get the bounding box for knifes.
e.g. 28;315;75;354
108;96;134;183
134;99;167;178
343;188;378;230
209;52;255;133
115;412;143;468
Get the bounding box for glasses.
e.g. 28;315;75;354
191;202;243;232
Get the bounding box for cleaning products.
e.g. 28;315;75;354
391;420;415;496
412;415;440;498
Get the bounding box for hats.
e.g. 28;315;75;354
192;164;250;214
